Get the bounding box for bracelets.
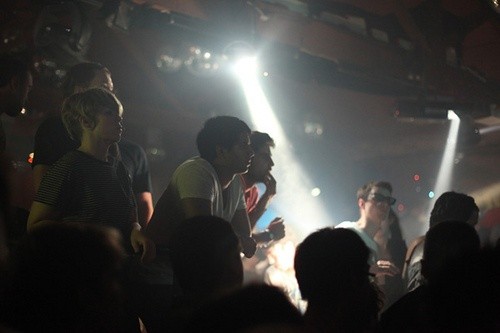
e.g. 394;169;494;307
265;228;276;243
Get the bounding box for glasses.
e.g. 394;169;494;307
367;192;396;205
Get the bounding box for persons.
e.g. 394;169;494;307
240;130;285;247
333;179;400;295
295;228;383;333
1;205;305;333
27;87;160;267
373;208;410;314
371;219;500;333
30;61;157;231
147;116;258;259
410;191;481;298
